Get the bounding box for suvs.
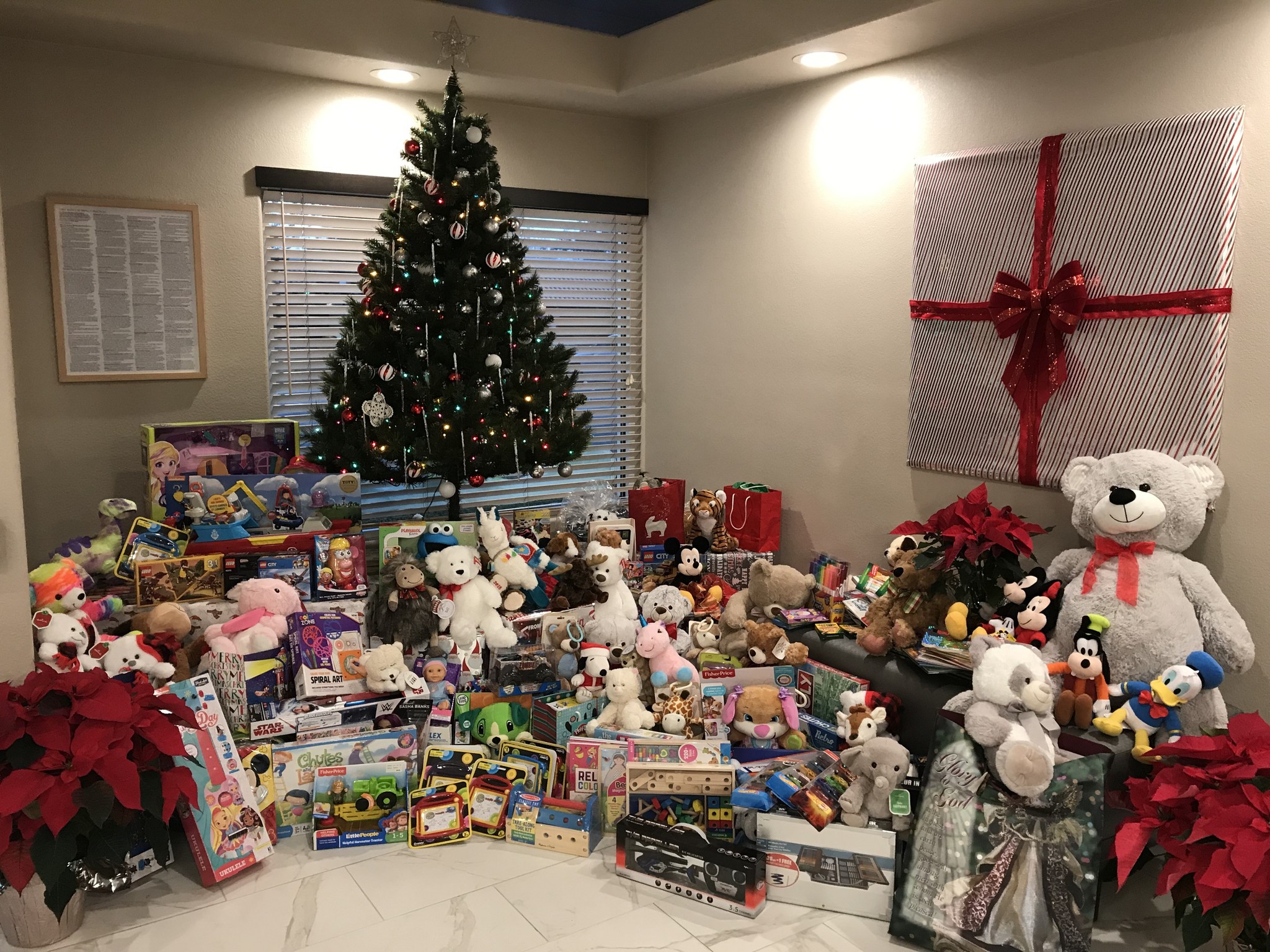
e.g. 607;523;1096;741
495;650;556;687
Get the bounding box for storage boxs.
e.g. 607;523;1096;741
118;415;902;917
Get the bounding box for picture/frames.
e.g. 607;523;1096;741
41;192;208;378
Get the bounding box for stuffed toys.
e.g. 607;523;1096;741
686;615;722;663
1006;579;1065;650
379;550;443;652
358;640;411;694
829;707;887;744
583;538;640;621
720;558;817;657
594;527;621;548
738;618;810;667
472;504;609;610
23;525;302;691
460;699;531;749
688;487;740;552
549;618;582;691
838;688;901;741
636;583;693;654
837;736;916;833
853;548;956;655
584;617;636;666
1029;444;1257;740
1049;612;1113;731
962;638;1062;799
646;692;701;741
881;532;920;568
573;641;610;704
945;563;1046;640
1101;651;1227;764
704;701;722;719
721;682;800;749
585;666;656;737
664;535;710;587
425;543;520;651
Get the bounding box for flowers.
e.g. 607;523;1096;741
885;478;1056;620
0;663;207;929
1106;709;1270;952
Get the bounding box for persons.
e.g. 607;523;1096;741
702;695;721;719
417;656;455;709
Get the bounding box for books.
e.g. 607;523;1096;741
901;630;974;676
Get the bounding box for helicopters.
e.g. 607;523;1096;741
630;845;699;883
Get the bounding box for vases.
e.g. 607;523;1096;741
1;869;86;948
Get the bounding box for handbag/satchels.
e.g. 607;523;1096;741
628;477;685;550
724;483;782;553
